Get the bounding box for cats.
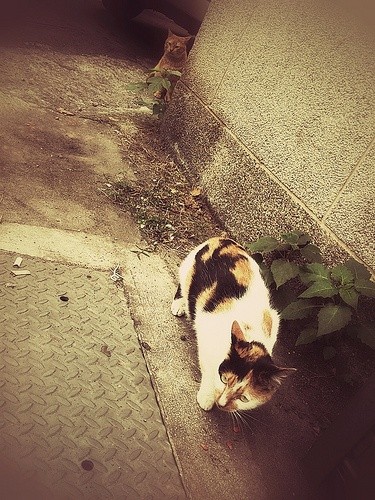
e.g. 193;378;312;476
171;235;298;437
